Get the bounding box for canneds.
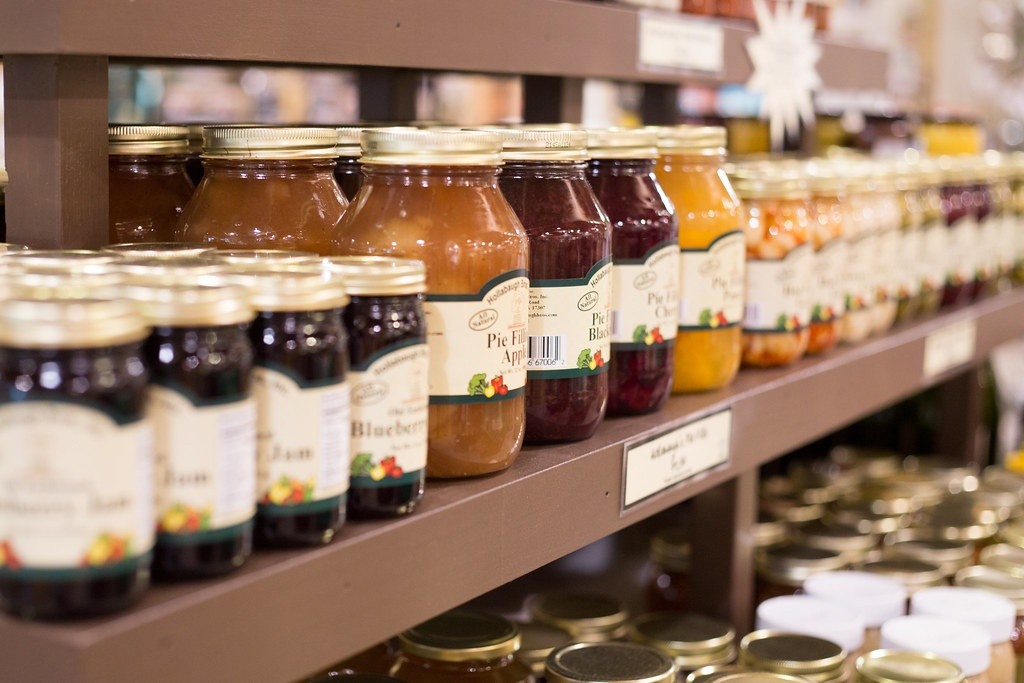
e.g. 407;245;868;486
0;121;1024;683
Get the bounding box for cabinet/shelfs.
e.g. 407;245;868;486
0;0;1024;683
